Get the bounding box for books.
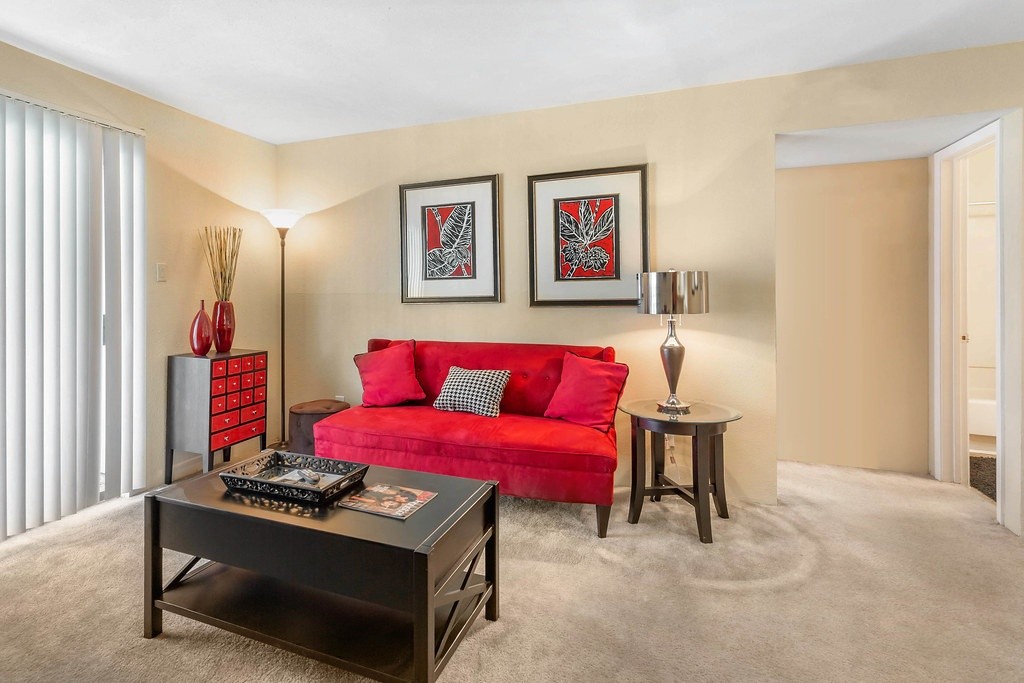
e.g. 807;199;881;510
275;469;349;489
338;482;438;521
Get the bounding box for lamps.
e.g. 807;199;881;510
260;208;306;453
637;268;709;411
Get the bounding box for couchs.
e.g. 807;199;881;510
313;339;618;538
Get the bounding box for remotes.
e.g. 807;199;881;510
298;468;321;484
286;480;320;488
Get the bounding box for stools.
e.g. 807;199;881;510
288;398;351;455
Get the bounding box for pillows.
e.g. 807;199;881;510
433;366;511;418
352;339;427;407
544;350;629;432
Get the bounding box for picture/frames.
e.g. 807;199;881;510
527;163;650;307
398;174;502;303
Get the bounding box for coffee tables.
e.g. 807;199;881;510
144;447;501;683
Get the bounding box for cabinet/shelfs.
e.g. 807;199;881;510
165;348;268;485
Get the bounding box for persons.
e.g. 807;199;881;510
351;484;417;509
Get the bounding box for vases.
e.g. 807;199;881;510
189;300;213;357
212;301;236;354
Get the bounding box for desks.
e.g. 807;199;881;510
617;398;743;543
